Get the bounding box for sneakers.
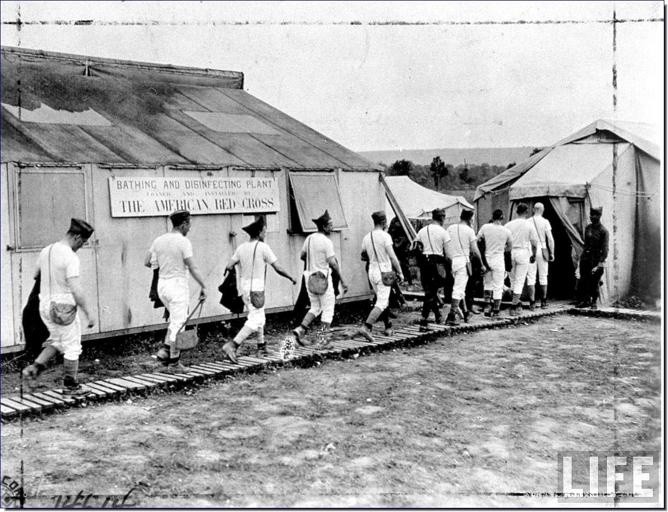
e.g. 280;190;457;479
22;300;597;398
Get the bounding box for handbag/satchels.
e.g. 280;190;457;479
382;272;398;286
437;264;446;278
308;271;327;295
543;249;549;262
466;262;472;276
250;291;264;308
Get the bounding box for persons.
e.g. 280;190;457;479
292;208;348;349
574;207;609;308
360;211;404;342
222;216;296;364
21;218;95;395
408;202;556;325
143;210;208;374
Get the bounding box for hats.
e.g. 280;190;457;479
71;204;602;239
